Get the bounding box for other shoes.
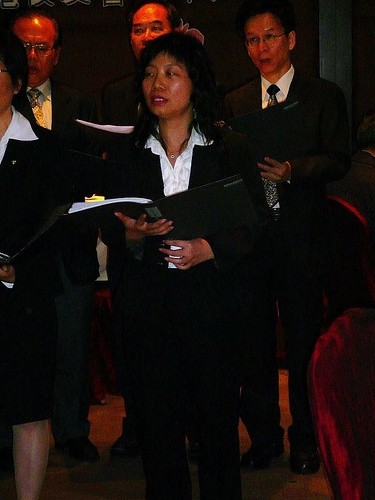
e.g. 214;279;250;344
241;441;284;469
64;435;97;464
110;434;140;458
289;443;320;475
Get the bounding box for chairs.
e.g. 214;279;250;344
306;307;375;500
328;195;375;308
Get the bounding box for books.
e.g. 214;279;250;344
227;97;329;162
59;174;258;238
61;119;134;159
0;201;74;267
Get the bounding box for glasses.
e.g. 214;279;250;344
245;33;287;49
23;43;54;57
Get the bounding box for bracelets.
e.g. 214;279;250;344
285;161;291;173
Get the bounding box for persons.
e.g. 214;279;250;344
103;2;183;475
0;20;84;500
97;31;276;500
14;12;106;470
328;116;375;230
221;7;352;476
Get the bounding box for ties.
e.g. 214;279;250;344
28;88;47;128
263;84;280;206
